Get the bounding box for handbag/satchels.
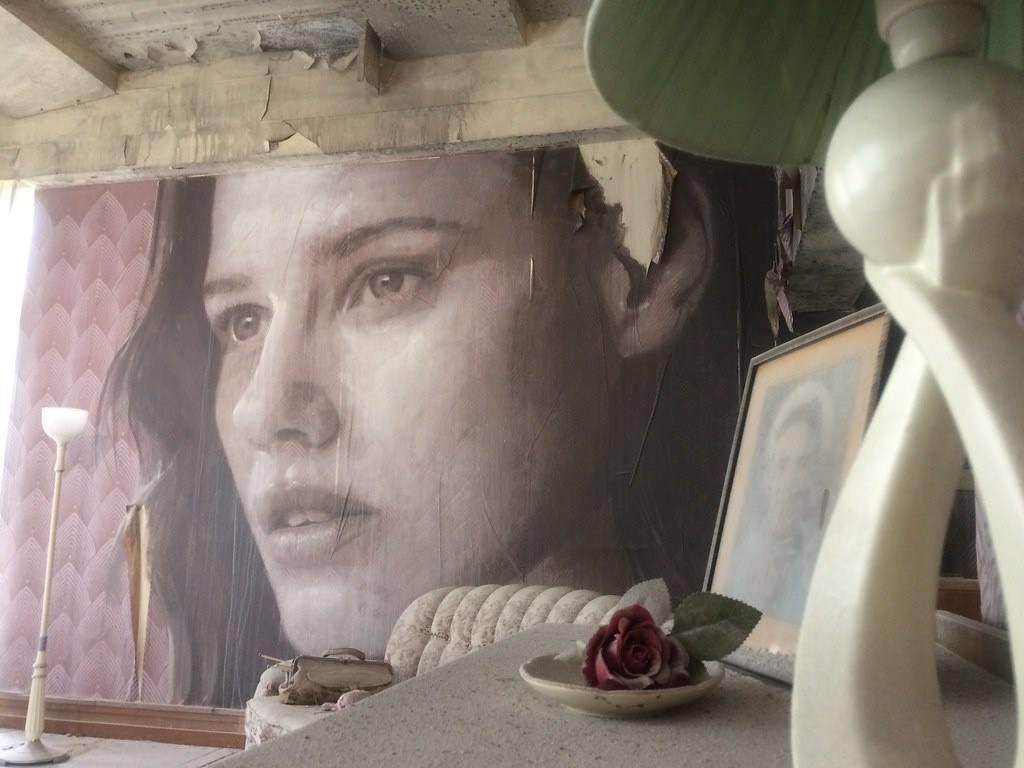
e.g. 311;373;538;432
279;649;394;705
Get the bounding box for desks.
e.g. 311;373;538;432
208;608;1018;768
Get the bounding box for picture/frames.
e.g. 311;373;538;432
691;302;907;694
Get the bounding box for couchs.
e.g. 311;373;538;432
244;582;680;753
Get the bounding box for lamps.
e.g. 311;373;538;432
0;405;93;768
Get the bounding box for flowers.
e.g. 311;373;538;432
562;576;763;689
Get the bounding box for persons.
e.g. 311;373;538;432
100;126;781;709
